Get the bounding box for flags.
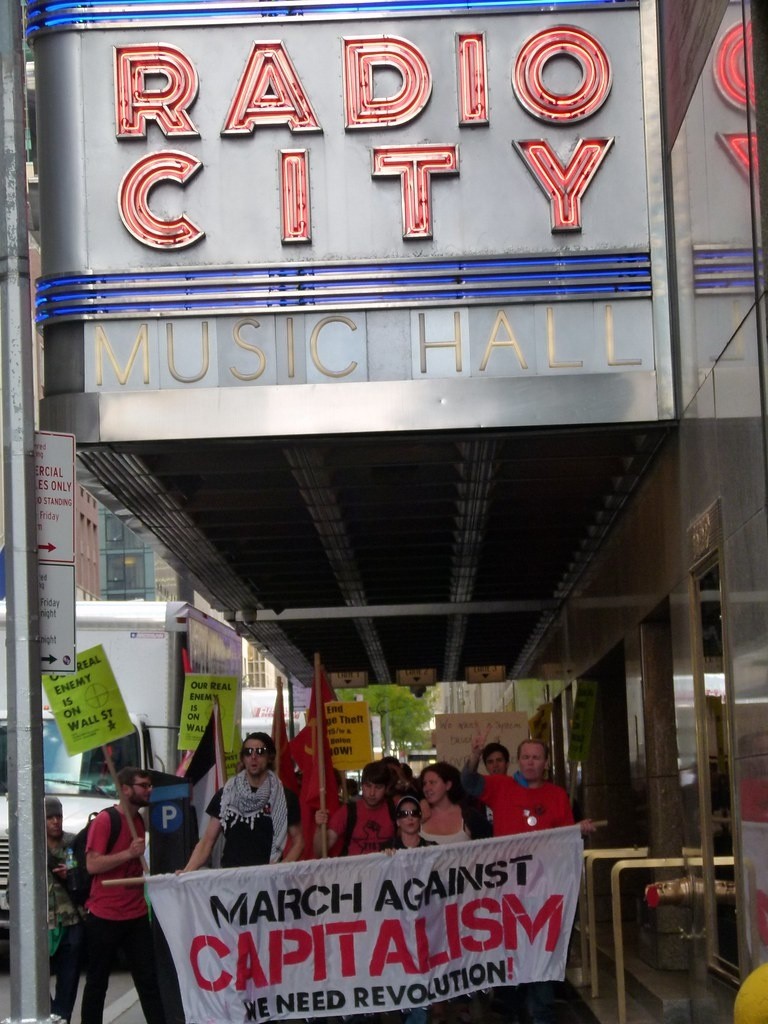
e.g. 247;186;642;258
145;672;344;872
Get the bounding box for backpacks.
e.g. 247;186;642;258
64;804;115;909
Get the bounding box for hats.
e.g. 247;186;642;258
45;796;63;815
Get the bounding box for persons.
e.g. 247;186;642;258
44;732;597;1024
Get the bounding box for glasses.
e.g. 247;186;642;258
396;809;421;819
134;783;153;788
242;747;267;756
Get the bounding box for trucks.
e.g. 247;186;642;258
1;593;244;976
241;686;306;776
